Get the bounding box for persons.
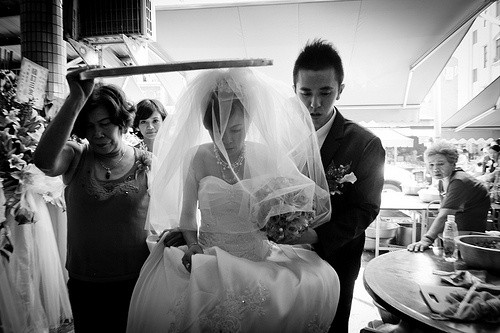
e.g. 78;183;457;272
127;72;340;333
407;143;492;252
256;36;386;333
32;66;186;333
484;144;500;203
132;98;168;153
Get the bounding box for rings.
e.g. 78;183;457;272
186;263;190;269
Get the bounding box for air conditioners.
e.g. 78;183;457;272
78;0;156;43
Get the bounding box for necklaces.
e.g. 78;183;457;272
99;152;125;179
214;144;246;184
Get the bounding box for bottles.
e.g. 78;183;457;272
443;214;458;263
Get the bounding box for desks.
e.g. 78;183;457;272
363;249;500;333
375;193;500;258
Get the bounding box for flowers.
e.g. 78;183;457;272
135;142;153;180
0;69;51;259
258;177;314;239
328;163;356;194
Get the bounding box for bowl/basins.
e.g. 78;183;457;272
455;235;500;271
417;191;441;203
364;220;399;251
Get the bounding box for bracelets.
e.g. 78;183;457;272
424;235;435;243
189;242;197;248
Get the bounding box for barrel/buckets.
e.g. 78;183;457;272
396;223;422;246
